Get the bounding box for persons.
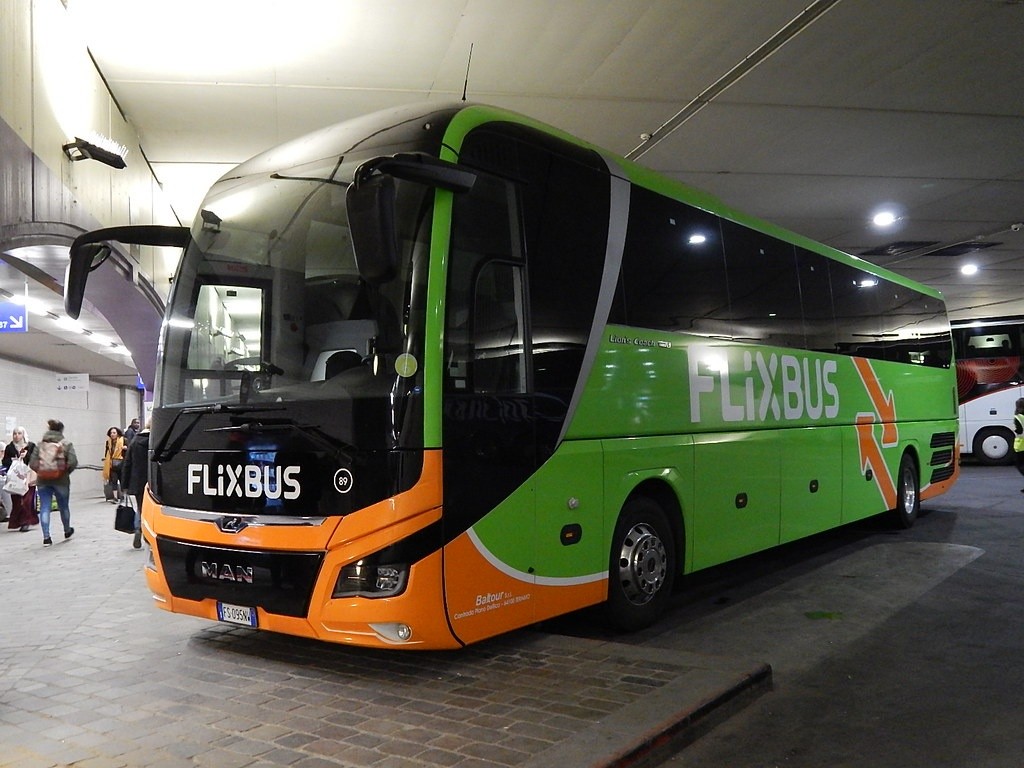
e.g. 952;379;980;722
125;418;140;444
114;417;152;549
0;426;40;532
27;419;74;547
1012;397;1024;493
102;427;130;504
0;441;13;522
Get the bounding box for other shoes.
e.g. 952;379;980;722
65;528;74;538
20;526;28;532
44;537;52;546
133;530;142;548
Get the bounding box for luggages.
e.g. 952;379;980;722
104;481;122;502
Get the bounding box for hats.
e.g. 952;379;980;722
48;420;64;431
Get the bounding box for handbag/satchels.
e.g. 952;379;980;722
115;495;136;534
112;458;123;471
2;456;38;496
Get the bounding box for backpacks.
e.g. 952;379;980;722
37;438;71;480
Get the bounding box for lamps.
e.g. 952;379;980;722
62;130;130;169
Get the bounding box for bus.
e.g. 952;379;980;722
951;314;1024;467
63;100;960;653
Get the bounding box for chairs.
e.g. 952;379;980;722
325;350;362;379
